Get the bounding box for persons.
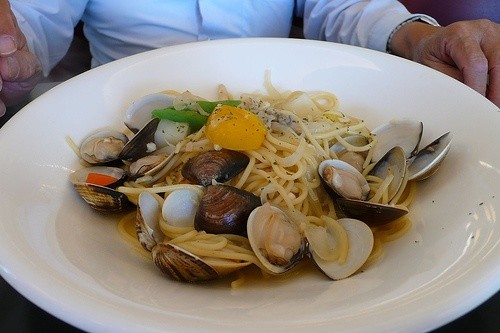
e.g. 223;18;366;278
0;1;499;128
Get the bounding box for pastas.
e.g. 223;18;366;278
66;70;456;288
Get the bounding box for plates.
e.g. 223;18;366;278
1;37;500;333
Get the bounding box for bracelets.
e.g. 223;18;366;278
386;18;439;54
